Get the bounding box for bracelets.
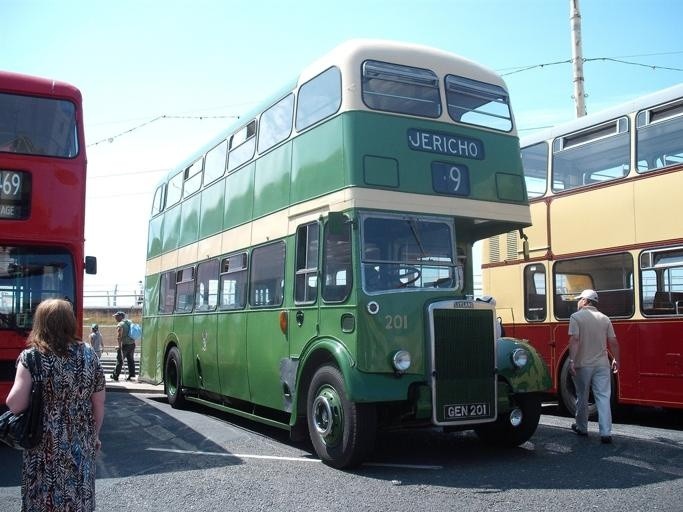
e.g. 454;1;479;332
569;359;574;363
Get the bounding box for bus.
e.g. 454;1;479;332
138;39;552;470
1;71;96;411
480;83;682;420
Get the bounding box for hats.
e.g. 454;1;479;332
92;323;98;331
113;311;125;317
573;289;599;303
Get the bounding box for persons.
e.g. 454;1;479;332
566;289;620;444
109;311;135;381
3;297;104;511
88;323;104;359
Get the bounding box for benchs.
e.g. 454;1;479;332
529;288;683;316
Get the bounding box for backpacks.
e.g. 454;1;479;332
124;319;142;340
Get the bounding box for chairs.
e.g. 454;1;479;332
582;153;683;187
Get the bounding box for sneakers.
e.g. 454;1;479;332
109;372;134;382
571;423;612;445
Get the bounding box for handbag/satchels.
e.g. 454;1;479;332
0;345;45;450
